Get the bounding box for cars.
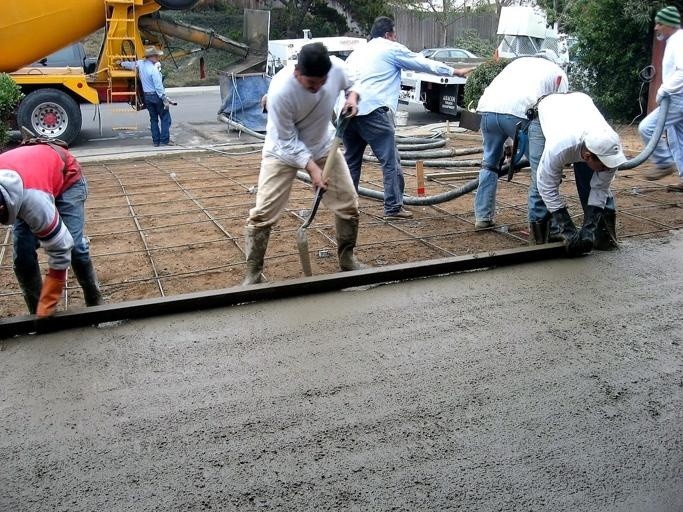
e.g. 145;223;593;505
420;48;491;78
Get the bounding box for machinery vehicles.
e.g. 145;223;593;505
0;0;271;147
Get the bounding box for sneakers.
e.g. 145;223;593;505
383;206;413;217
159;140;175;146
643;165;676;181
153;143;159;147
666;182;683;192
475;219;495;229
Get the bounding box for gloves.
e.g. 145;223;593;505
551;207;581;258
162;100;170;111
572;205;604;254
35;274;67;319
115;60;122;67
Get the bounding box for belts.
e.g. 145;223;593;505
143;92;157;95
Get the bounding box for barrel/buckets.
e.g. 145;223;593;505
395;111;409;126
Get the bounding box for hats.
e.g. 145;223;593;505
142;46;163;57
534;49;558;64
654;6;681;29
584;128;628;169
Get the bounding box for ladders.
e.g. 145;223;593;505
103;0;138;132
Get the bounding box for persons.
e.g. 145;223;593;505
333;17;476;221
0;144;103;325
115;46;171;147
242;42;373;285
527;92;628;257
637;6;683;192
475;49;569;242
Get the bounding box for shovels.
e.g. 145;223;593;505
297;106;353;276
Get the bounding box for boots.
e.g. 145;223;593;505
335;213;372;271
242;226;270;286
